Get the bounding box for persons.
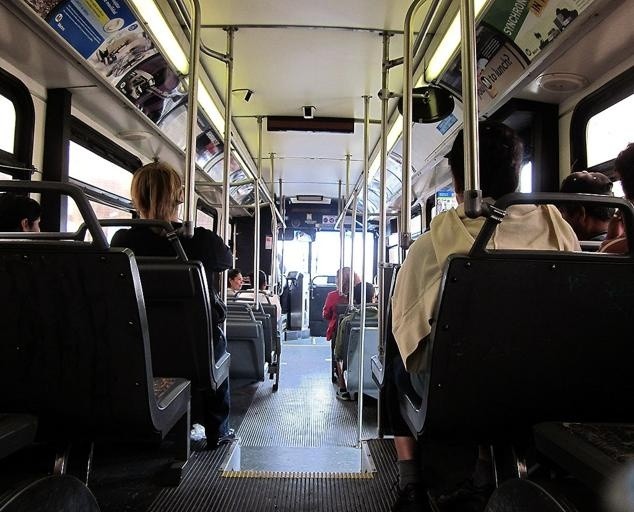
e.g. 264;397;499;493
379;120;634;512
322;267;381;401
110;161;236;449
276;265;289;331
227;268;282;331
0;192;43;232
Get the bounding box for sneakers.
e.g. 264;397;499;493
392;481;431;510
337;387;350;401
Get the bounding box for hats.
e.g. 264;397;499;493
444;121;521;160
559;171;614;194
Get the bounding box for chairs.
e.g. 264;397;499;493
74;218;230;456
223;279;278;383
480;477;582;511
331;302;380;403
395;192;632;510
0;179;194;509
0;412;100;508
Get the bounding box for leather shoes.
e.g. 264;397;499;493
208;428;235;449
